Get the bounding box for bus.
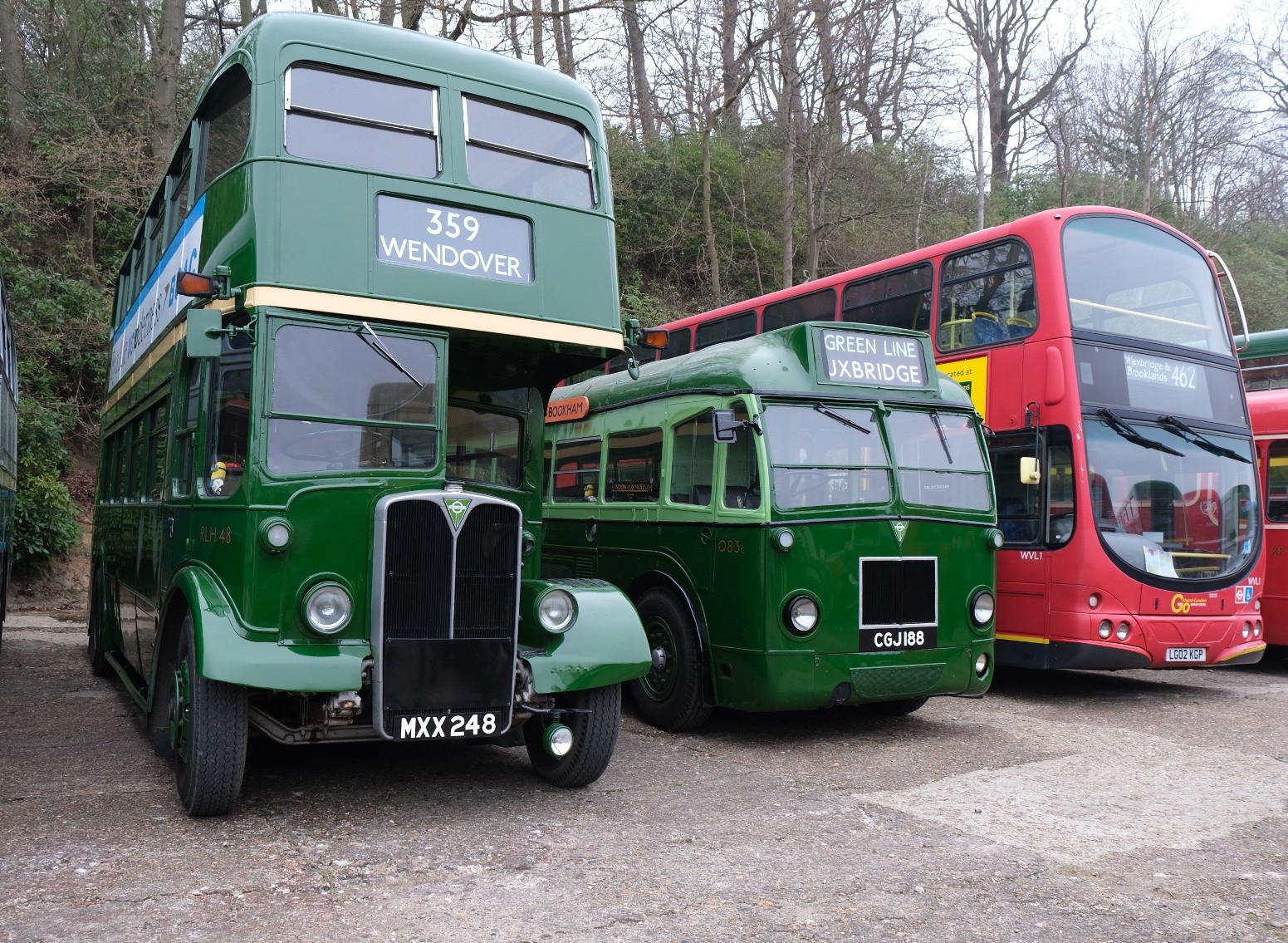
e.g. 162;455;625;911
0;268;20;640
84;13;655;821
440;204;1288;733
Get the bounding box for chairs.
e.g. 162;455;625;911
998;498;1035;538
694;484;754;509
971;312;1034;346
